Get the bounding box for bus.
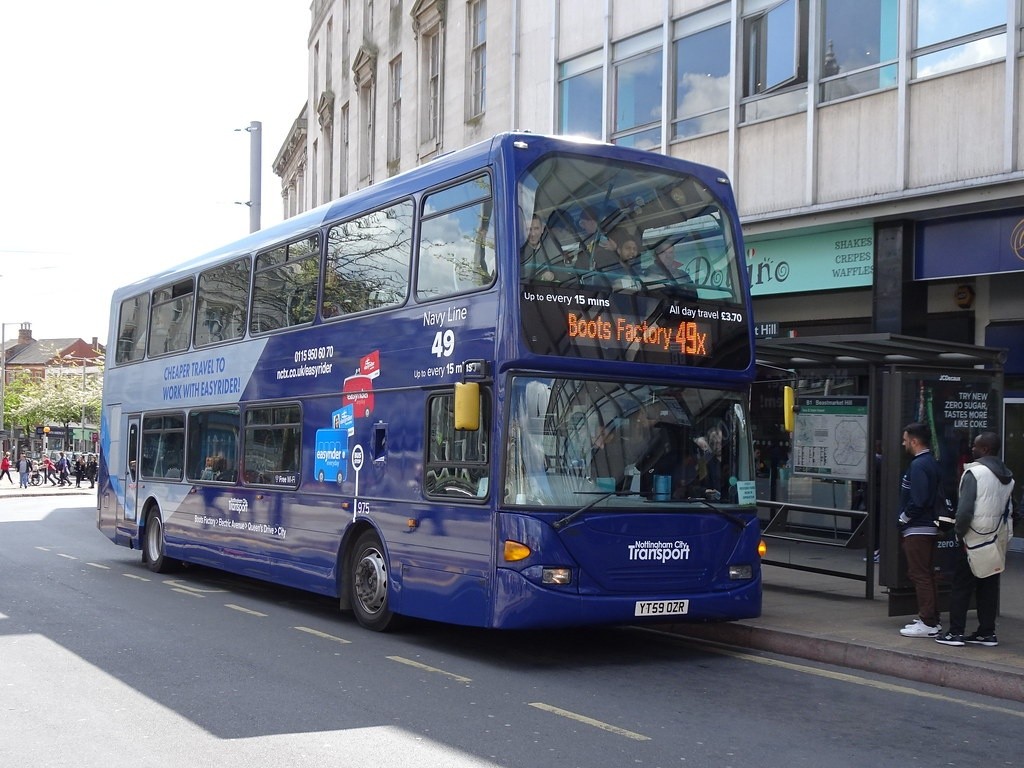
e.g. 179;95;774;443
93;130;798;635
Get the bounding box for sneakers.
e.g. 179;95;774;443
905;619;942;630
935;631;965;645
899;619;939;637
964;632;998;645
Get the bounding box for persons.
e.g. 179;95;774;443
896;423;946;636
512;204;723;497
934;431;1015;646
863;439;883;563
0;450;99;489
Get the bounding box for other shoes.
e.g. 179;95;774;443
58;483;65;487
25;486;27;489
52;483;56;486
68;482;72;486
20;486;22;488
89;487;94;488
58;479;61;484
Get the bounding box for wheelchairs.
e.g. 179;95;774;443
26;468;44;486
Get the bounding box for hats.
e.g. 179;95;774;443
580;206;601;220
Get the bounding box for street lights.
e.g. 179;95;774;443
43;427;51;484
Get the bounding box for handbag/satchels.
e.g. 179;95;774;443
962;514;1008;578
932;499;956;557
